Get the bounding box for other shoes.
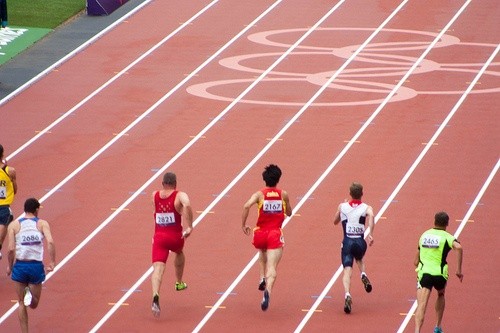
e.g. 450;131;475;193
361;274;372;293
261;290;269;310
344;295;351;313
258;277;266;291
23;287;33;306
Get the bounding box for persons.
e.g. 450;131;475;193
334;183;374;313
152;173;193;318
7;198;56;333
0;144;17;259
414;212;463;332
241;164;292;310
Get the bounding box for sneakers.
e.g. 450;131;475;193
434;326;443;333
152;293;160;317
175;281;187;291
